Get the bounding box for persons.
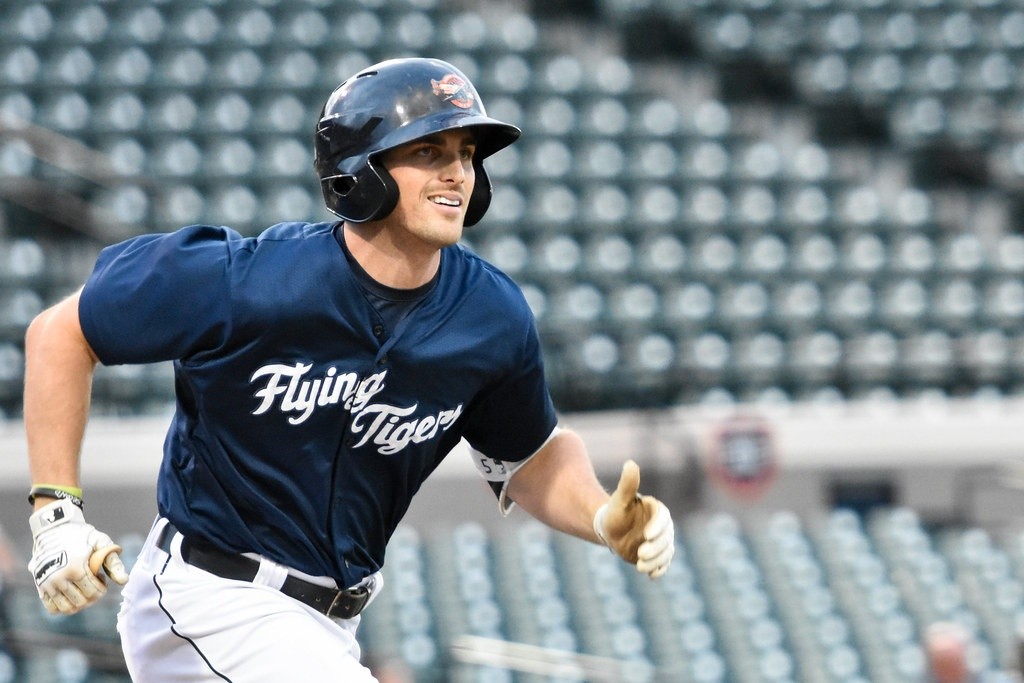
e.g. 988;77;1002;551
916;621;1015;683
23;51;676;683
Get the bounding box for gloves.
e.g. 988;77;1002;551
27;498;142;632
593;460;675;580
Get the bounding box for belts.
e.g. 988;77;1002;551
153;516;376;621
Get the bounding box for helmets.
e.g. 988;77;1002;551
313;57;521;228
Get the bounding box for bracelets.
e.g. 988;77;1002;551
28;483;84;512
